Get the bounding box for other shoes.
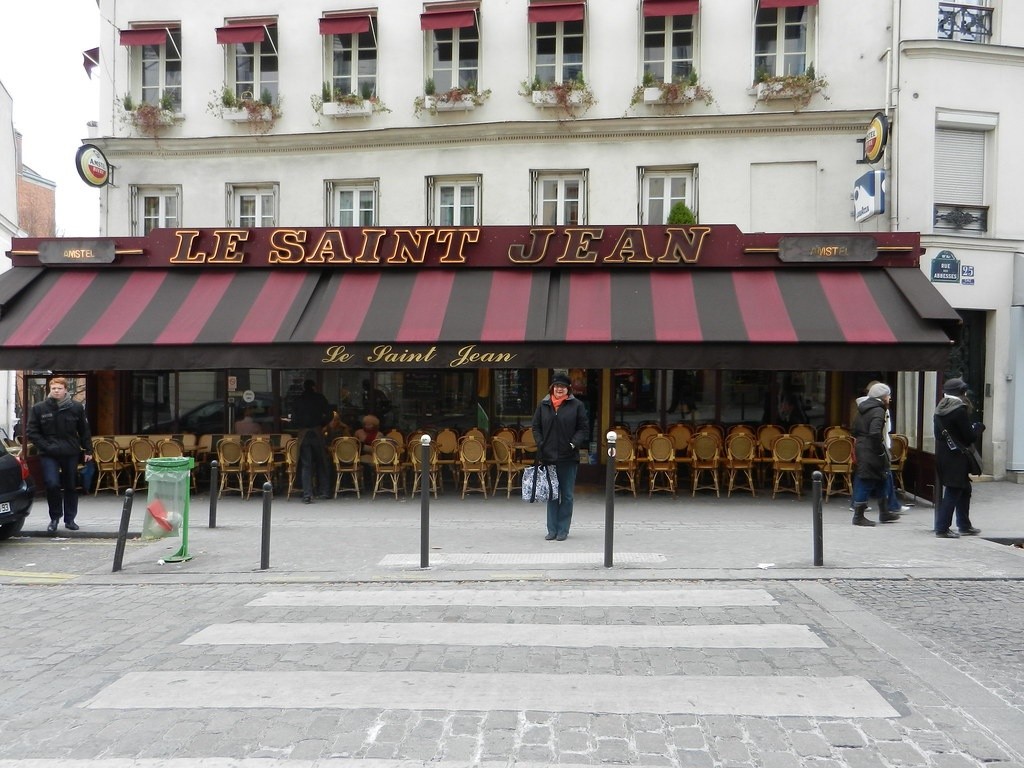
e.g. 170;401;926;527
304;497;310;504
889;505;910;514
322;492;332;499
849;507;872;512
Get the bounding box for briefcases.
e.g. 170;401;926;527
80;459;97;493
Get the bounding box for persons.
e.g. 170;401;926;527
663;369;697;414
532;376;588;542
850;377;986;538
28;378;93;533
234;376;392;504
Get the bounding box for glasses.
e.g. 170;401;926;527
554;384;568;388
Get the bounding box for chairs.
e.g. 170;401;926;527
2;425;908;501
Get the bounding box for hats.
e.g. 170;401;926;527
942;378;966;390
550;372;572;391
868;383;891;397
363;415;379;425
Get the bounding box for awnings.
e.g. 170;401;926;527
0;224;963;369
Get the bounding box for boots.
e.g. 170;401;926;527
853;508;876;526
878;503;900;522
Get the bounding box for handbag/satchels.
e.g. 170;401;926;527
522;449;559;503
965;443;984;475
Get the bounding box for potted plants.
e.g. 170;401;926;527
750;61;831;115
519;71;600;130
220;85;282;135
620;63;720;120
413;76;491;118
122;91;177;149
318;79;392;119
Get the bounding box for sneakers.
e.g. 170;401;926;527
936;530;960;538
958;527;980;536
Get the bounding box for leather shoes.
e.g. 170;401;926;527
65;521;79;530
48;519;59;532
545;534;567;541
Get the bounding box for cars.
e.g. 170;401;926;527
135;392;295;433
0;428;33;540
